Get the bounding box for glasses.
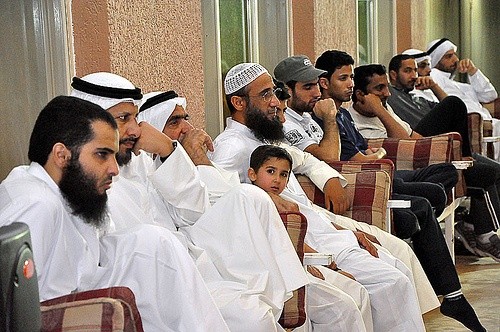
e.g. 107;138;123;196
248;88;282;102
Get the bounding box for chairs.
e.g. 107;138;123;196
34;99;500;332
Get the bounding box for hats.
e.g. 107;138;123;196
274;54;328;83
224;63;268;95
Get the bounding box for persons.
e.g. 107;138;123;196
0;96;231;332
272;39;499;332
70;63;428;332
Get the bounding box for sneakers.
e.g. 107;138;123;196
473;234;500;262
454;220;477;256
